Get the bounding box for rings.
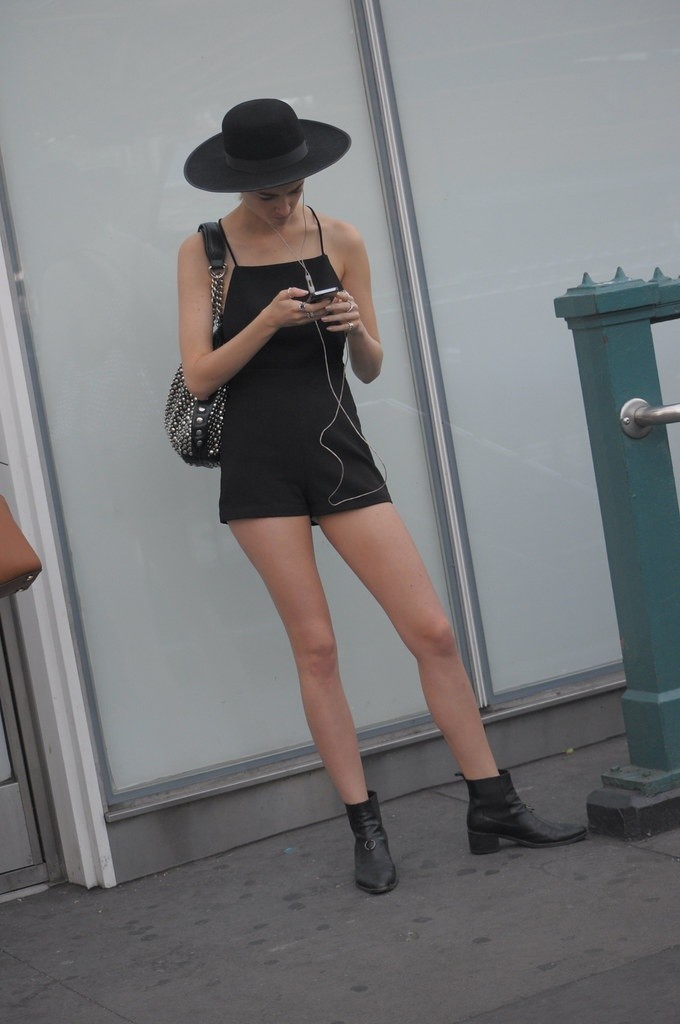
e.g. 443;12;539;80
288;288;294;298
346;321;353;330
347;300;353;313
299;302;305;311
305;312;313;318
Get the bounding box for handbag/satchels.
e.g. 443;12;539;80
163;222;228;467
0;494;42;598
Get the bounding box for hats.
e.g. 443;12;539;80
183;98;352;192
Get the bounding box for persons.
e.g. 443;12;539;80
178;98;585;894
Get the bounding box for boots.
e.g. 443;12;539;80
345;790;398;893
454;769;587;854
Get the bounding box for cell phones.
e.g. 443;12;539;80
306;287;339;304
